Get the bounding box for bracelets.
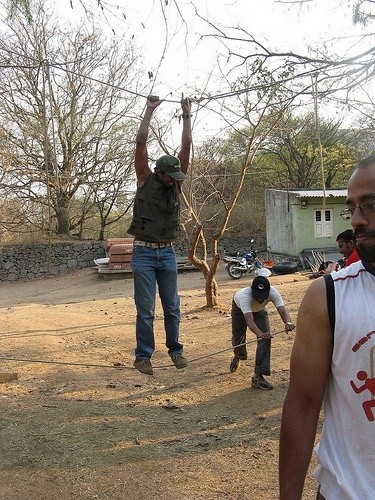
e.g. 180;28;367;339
182;114;192;119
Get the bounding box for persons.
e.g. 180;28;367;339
280;155;375;500
230;276;294;389
318;229;360;276
126;93;192;375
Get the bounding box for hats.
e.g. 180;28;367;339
154;155;188;179
250;276;270;299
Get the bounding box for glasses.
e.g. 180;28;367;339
339;199;375;221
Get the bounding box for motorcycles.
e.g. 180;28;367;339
221;238;263;279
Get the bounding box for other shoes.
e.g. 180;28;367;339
229;356;239;372
170;353;188;369
250;375;273;390
132;357;153;375
